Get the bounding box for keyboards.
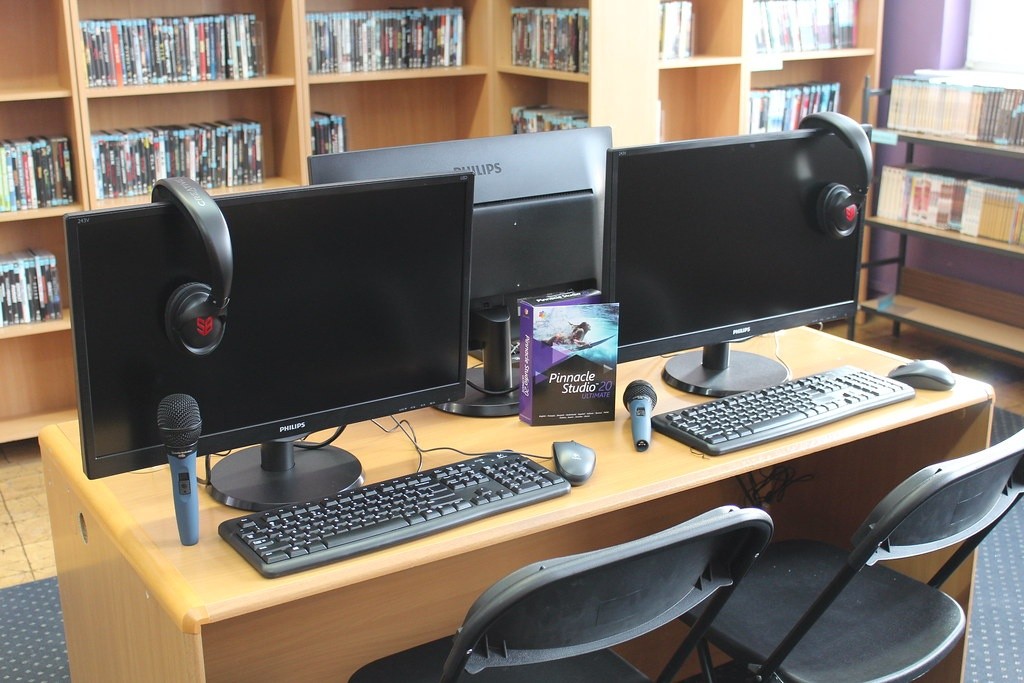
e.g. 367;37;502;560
218;449;572;579
650;366;915;457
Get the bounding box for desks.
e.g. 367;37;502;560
39;326;996;683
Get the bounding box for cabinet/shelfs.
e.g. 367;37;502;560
492;0;660;149
70;0;310;210
298;0;492;188
660;0;886;326
848;70;1024;370
0;0;91;445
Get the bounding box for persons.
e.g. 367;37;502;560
542;322;592;350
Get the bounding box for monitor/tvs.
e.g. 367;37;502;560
64;125;872;513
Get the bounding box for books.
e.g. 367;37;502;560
310;109;348;156
89;117;265;202
511;101;588;136
1;246;63;329
510;6;590;74
884;67;1024;146
0;135;74;214
751;1;859;56
875;161;1024;248
659;0;696;60
304;6;465;76
750;79;842;137
78;11;260;89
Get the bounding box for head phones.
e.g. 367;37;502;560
799;111;874;239
150;176;233;356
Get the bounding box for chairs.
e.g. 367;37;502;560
681;427;1024;683
348;504;775;683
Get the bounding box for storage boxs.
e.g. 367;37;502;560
517;288;619;427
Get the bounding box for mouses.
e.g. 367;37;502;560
888;360;955;391
550;441;596;486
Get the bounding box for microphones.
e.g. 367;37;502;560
623;379;657;452
158;393;202;546
